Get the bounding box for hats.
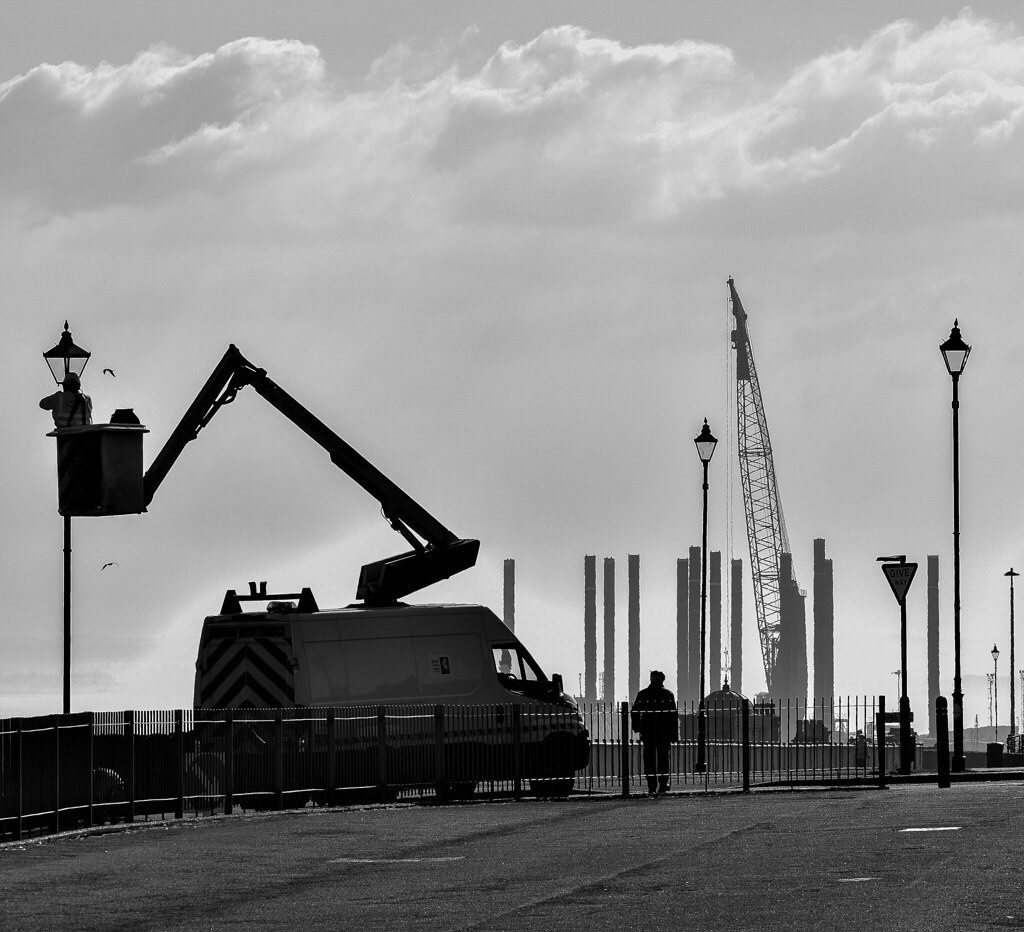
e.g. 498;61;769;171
650;671;665;680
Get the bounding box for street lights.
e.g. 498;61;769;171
692;421;719;773
42;319;91;716
989;643;999;743
1005;565;1021;735
937;317;973;773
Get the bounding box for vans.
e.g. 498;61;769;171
190;602;594;807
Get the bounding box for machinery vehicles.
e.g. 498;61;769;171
42;345;482;610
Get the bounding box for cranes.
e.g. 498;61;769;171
717;273;810;706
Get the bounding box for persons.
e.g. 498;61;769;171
630;670;679;794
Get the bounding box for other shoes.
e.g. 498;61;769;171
659;784;670;791
648;789;656;793
